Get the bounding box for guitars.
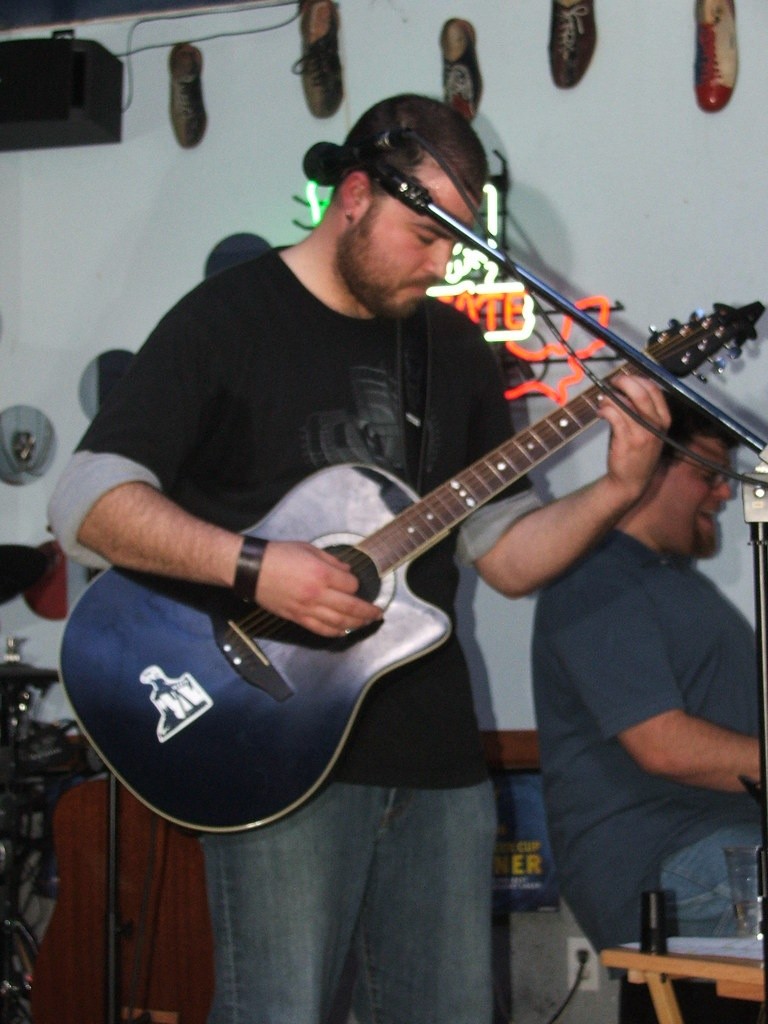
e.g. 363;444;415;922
59;300;765;837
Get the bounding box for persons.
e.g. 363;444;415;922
528;391;768;962
43;95;672;1024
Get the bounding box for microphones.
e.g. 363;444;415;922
302;130;406;186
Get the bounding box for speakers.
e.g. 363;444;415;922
0;39;123;152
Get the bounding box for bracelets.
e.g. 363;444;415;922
229;534;268;604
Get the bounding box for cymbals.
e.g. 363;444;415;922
1;543;45;606
1;662;57;681
